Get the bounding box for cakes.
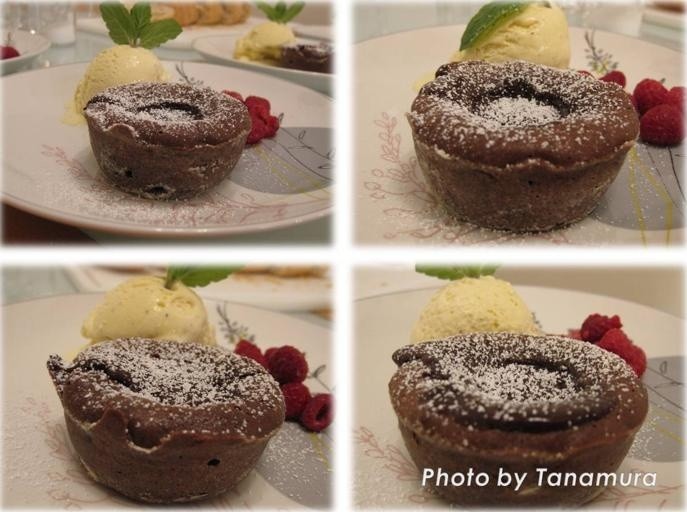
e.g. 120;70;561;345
383;330;649;509
407;54;643;233
84;80;252;203
47;337;287;506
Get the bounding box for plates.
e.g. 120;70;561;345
1;1;336;81
1;292;334;509
641;1;686;27
356;284;683;507
1;60;334;241
353;24;686;241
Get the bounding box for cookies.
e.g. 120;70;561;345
151;1;251;27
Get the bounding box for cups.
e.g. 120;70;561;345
578;0;644;36
